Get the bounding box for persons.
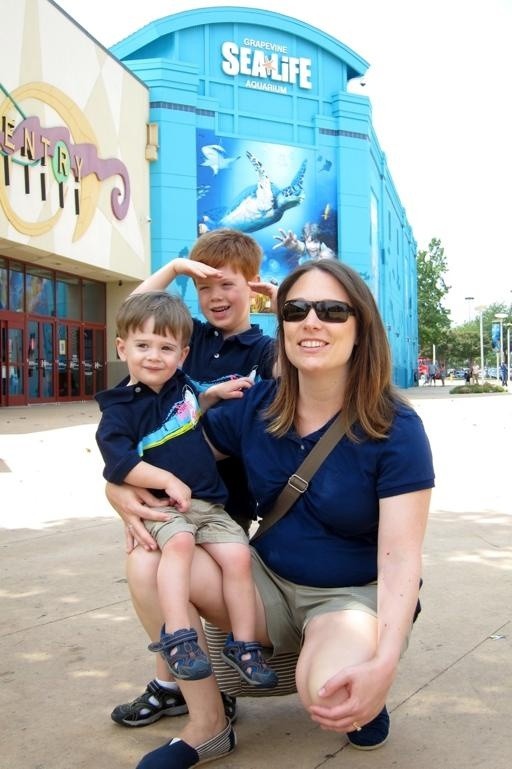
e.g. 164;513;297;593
95;289;280;691
106;260;435;769
271;223;337;264
111;230;282;727
419;361;508;387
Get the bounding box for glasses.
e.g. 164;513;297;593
281;299;355;323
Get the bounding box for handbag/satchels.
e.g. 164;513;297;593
204;620;300;697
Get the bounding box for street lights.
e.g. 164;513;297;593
475;303;512;386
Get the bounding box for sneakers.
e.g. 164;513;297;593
147;623;212;680
136;715;237;768
220;692;236;723
111;679;188;728
220;630;278;688
346;705;391;751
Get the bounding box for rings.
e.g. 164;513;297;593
352;721;362;733
